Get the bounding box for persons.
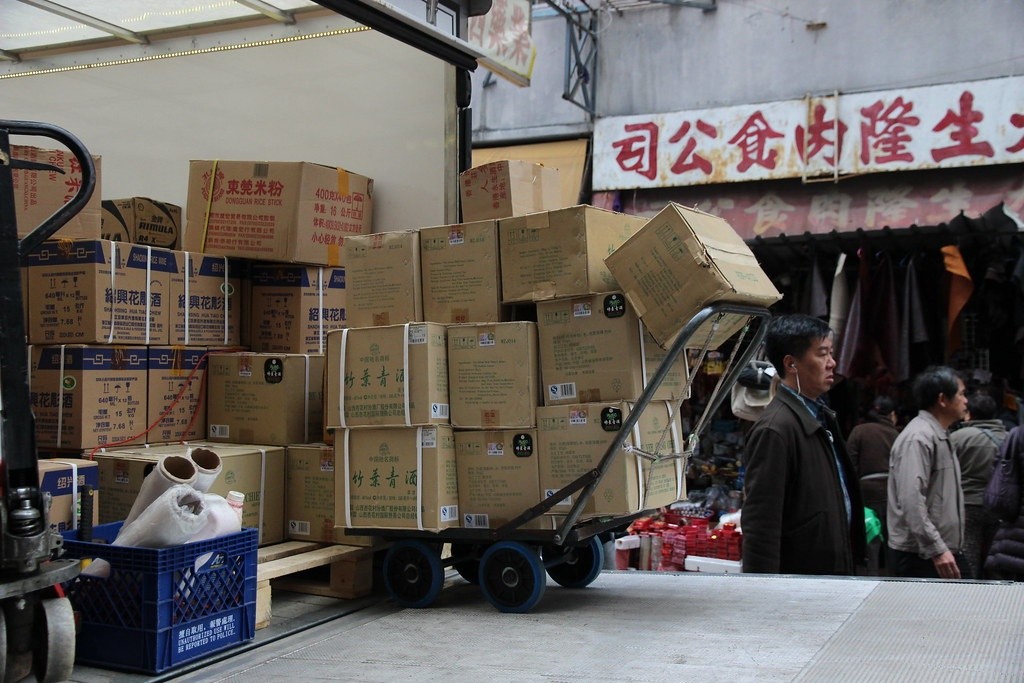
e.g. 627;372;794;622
740;314;869;575
845;365;1024;582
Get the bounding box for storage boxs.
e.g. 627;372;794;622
604;202;783;354
4;137;690;676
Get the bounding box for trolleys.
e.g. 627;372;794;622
0;118;96;682
344;298;775;618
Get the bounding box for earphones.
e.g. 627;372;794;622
791;364;796;369
